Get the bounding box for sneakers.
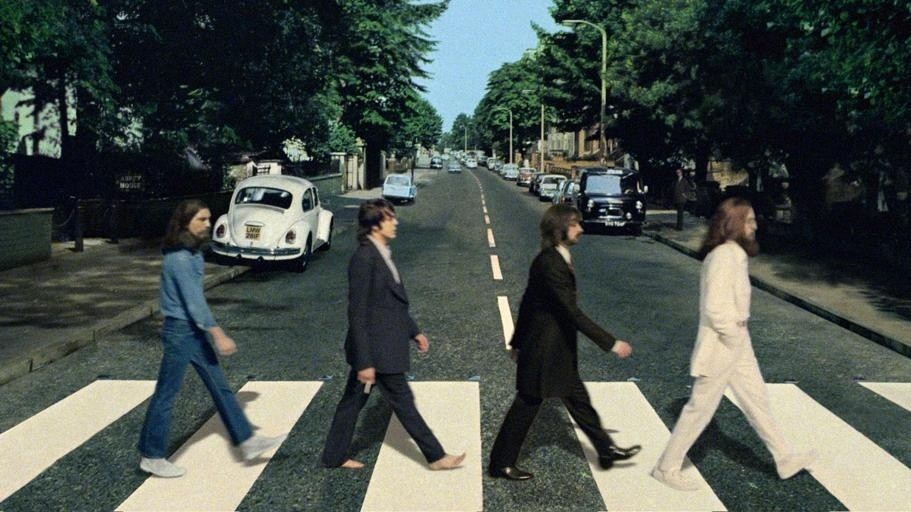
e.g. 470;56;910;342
651;468;697;491
777;447;821;480
140;457;185;478
241;433;287;461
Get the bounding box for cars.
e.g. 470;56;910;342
381;174;418;205
430;152;487;175
486;157;579;204
209;175;337;274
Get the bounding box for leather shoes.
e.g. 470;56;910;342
598;446;640;469
489;467;534;480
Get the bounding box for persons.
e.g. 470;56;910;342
486;205;640;482
674;168;689;231
137;200;285;479
321;198;466;472
650;200;817;488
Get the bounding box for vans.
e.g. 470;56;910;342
580;169;648;238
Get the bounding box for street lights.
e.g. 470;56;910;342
460;125;469;156
525;88;545;172
496;107;514;164
562;18;608;160
